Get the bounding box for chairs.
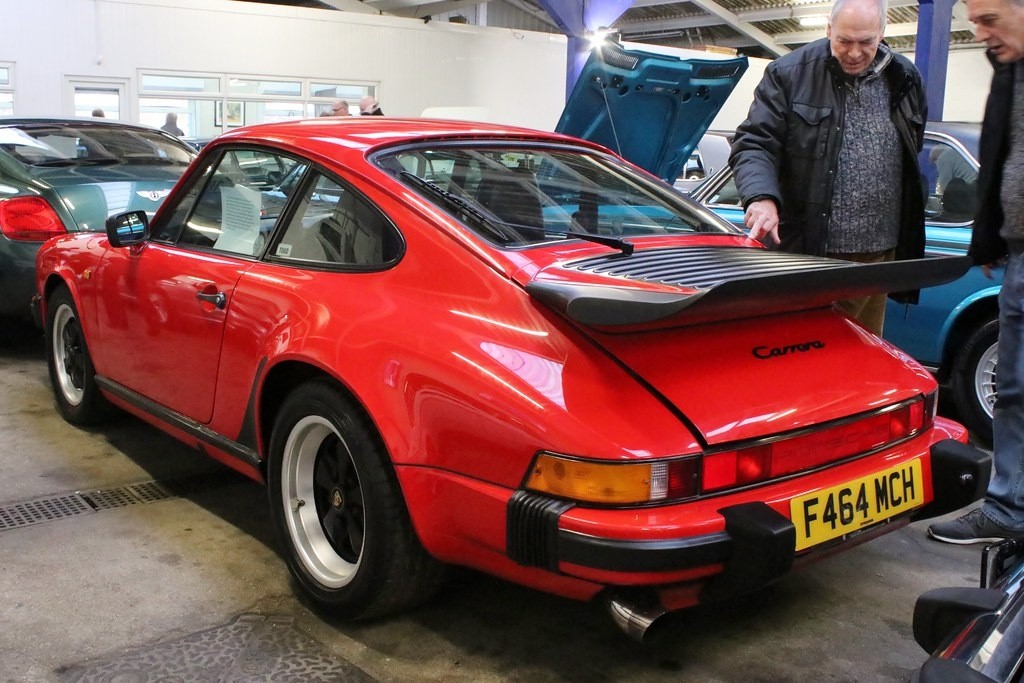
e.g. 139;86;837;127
286;167;543;260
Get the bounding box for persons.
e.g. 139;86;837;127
928;146;979;214
359;97;383;116
92;110;105;116
160;112;184;137
929;3;1024;545
725;2;927;352
332;101;350;116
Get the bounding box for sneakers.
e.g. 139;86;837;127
928;507;1024;544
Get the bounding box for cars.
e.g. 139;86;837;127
535;34;1008;452
0;112;239;335
33;119;973;646
